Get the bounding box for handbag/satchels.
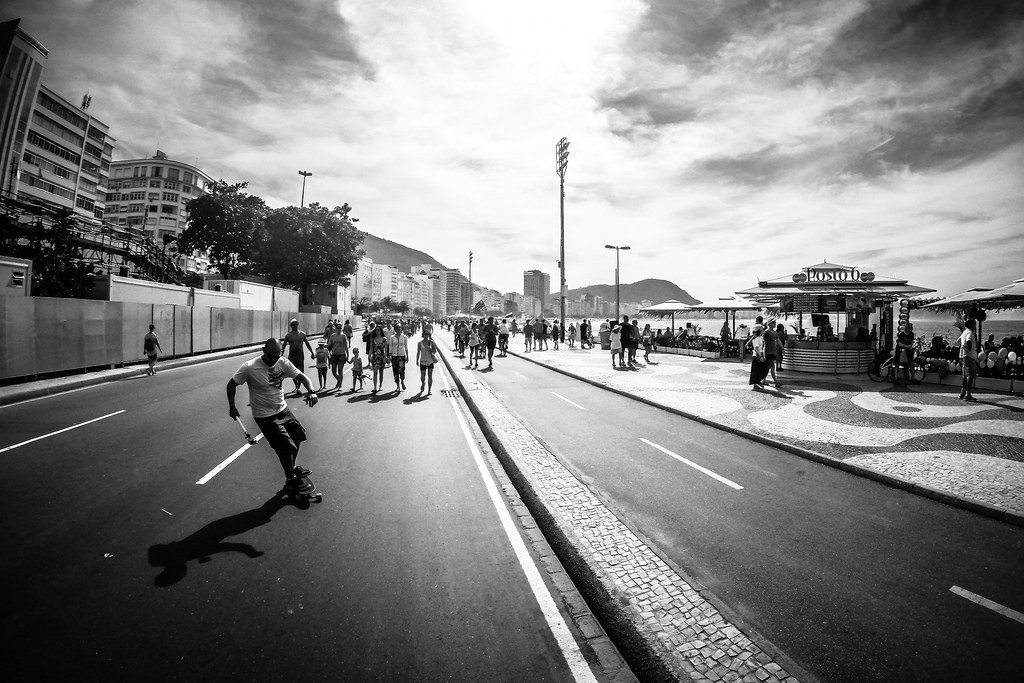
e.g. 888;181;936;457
431;353;438;363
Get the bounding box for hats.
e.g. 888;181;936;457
318;338;325;344
290;319;298;325
612;325;622;332
753;324;765;331
767;317;775;326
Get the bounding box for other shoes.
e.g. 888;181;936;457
754;384;764;390
286;475;311;491
291;385;432;396
152;373;156;375
775;382;783;387
963;395;979;401
146;369;151;376
960;394;965;397
763;382;770;386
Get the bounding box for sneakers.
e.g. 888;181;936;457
293;466;312;477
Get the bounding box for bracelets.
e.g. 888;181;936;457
230;406;235;408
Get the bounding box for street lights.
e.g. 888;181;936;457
555;136;570;338
604;245;632;322
299;169;313;208
467;251;477;310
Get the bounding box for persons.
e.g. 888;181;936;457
656;322;696;346
919;334;1024;376
749;316;788;389
984;334;1001;350
227;338;318;491
580;319;597;349
958;320;978;401
511;319;518;337
143;324;162;376
522;319;581;352
313;317;438;395
434;317;510;368
609;314;654;368
720;322;731;358
281;318;314;395
893;323;918;384
599;319;611;332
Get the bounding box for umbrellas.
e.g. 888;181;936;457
684;294;762;338
634;295;696;335
918;278;1024;352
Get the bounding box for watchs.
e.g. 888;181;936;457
308;390;316;395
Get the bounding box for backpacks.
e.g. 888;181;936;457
145;338;154;352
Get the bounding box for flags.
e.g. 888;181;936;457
474;299;485;311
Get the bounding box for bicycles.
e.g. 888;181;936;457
478;337;488;359
868;344;927;384
498;336;507;357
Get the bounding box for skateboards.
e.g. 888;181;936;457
292;465;323;502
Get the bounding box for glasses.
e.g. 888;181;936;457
337;328;342;329
425;333;429;335
292;324;298;326
374;329;379;331
264;347;281;359
